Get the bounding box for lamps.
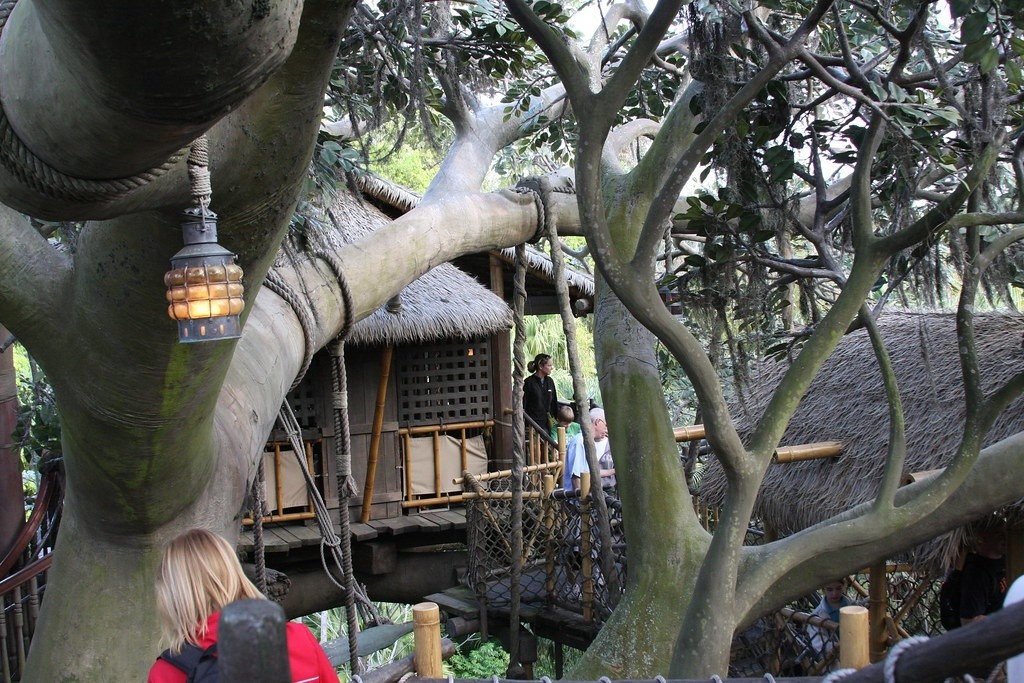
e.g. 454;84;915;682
165;222;248;343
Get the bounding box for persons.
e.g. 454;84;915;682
550;406;580;490
148;530;342;683
563;407;623;603
940;527;1008;682
521;353;564;470
806;576;859;674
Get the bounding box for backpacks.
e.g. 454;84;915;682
155;638;221;683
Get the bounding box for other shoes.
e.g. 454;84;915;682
591;567;608;586
563;583;583;603
